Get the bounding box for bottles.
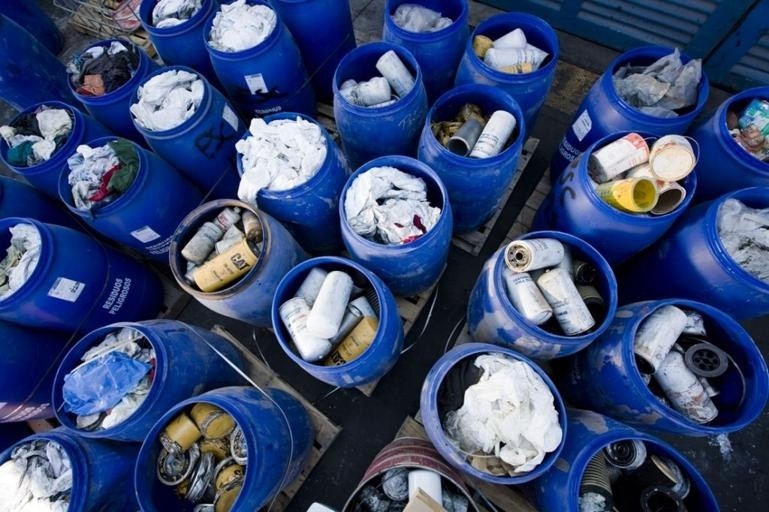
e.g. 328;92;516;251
407;469;443;509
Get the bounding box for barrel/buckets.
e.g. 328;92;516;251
552;299;719;444
178;70;235;193
237;113;355;247
269;255;402;388
338;154;453;288
180;200;313;336
419;84;526;235
332;48;432;163
210;47;317;112
549;131;698;265
468;232;619;362
453;47;559;125
554;50;711;175
420;342;567;412
